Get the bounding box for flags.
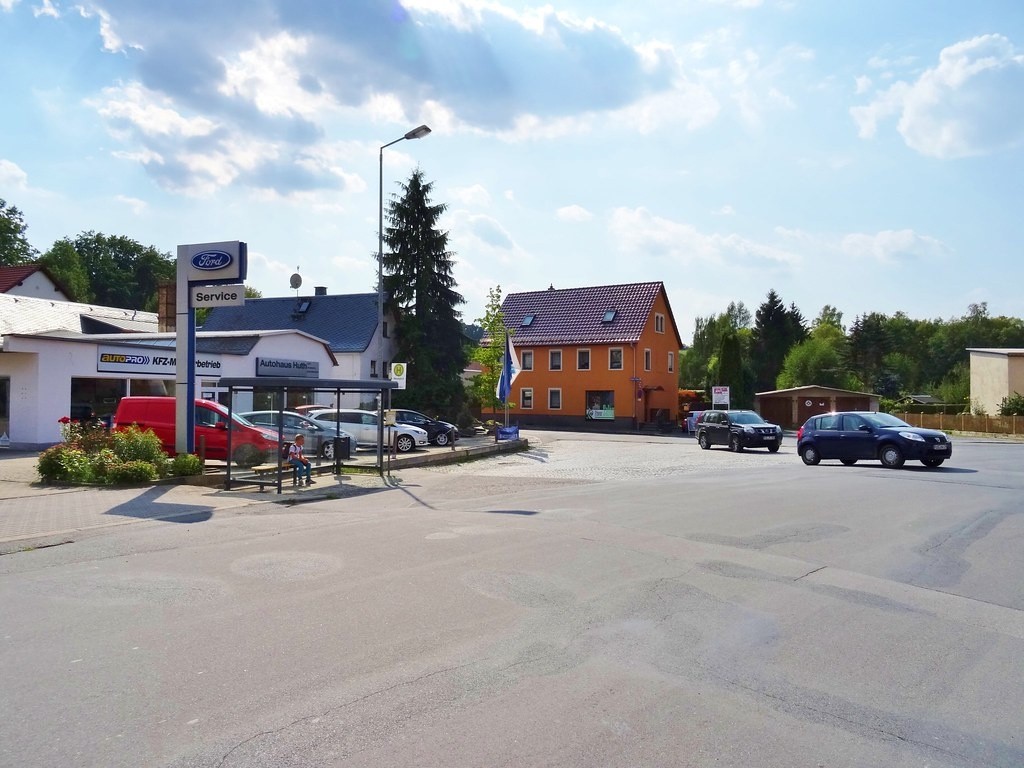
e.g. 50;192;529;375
496;335;522;404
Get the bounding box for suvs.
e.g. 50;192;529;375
796;411;953;470
694;409;783;453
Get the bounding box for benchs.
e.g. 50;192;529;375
251;464;298;493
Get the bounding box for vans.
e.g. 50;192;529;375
111;396;286;468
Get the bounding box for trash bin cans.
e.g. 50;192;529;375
332;436;350;461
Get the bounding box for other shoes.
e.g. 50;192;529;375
298;480;303;485
305;479;316;484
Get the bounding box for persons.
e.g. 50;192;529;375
288;435;316;485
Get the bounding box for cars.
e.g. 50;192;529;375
293;405;337;427
302;409;429;453
361;408;460;448
80;404;116;432
231;410;358;459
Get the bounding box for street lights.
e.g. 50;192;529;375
377;124;432;470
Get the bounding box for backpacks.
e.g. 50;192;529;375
282;442;301;459
